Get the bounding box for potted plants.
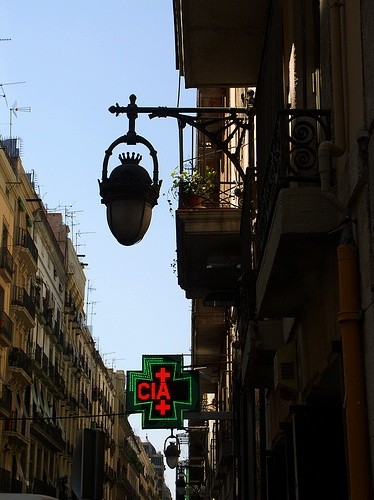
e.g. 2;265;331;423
171;161;217;206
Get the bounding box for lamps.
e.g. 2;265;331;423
175;466;204;496
165;412;234;469
97;93;255;246
